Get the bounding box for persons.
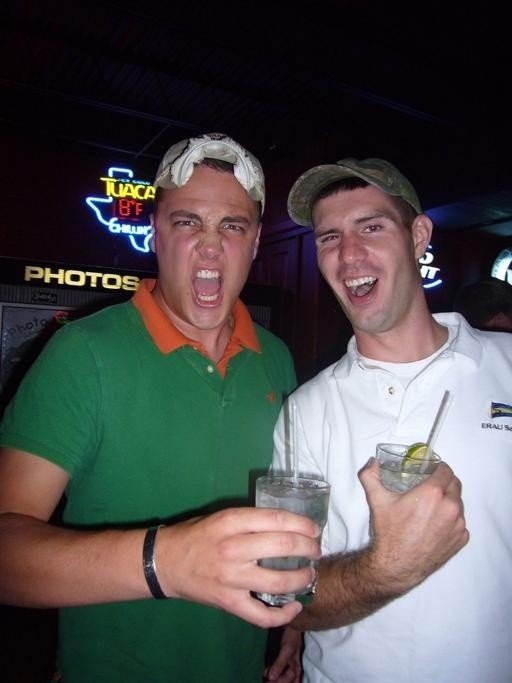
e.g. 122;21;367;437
452;277;512;331
1;130;321;683
255;158;512;683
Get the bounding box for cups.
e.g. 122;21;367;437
254;476;331;604
375;444;443;501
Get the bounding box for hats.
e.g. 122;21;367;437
153;133;266;215
285;159;421;228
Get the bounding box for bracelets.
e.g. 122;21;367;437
143;523;168;600
